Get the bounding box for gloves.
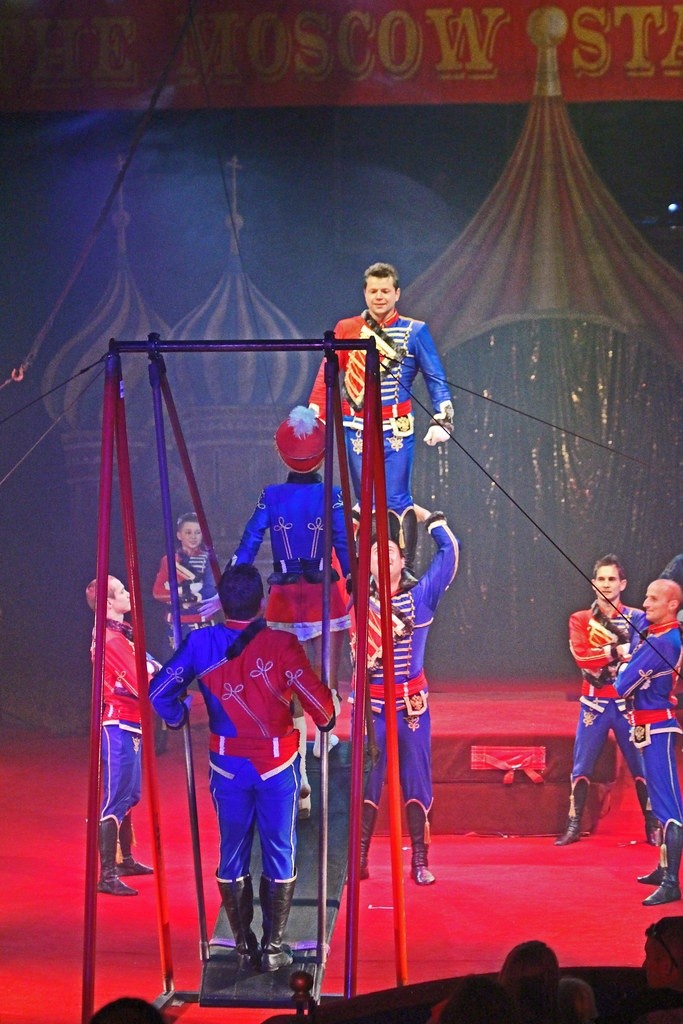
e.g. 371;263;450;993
414;502;431;524
331;689;340;717
346;592;380;617
621;643;631;653
146;662;155;674
423;425;450;446
353;503;376;515
619;663;628;671
178;581;203;595
184;694;193;710
198;594;223;618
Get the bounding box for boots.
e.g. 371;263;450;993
553;774;590;845
98;809;154;895
352;507;418;592
345;800;377;884
637;819;683;905
635;778;662;846
216;867;298;972
405;798;436;884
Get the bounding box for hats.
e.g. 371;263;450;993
277;405;329;472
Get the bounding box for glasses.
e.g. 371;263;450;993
651;920;679;969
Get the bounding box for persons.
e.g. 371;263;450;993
432;914;683;1024
152;513;222;757
91;996;164;1023
85;575;162;896
306;263;452;597
148;564;340;980
554;554;661;849
333;500;461;884
234;404;358;820
611;580;683;905
